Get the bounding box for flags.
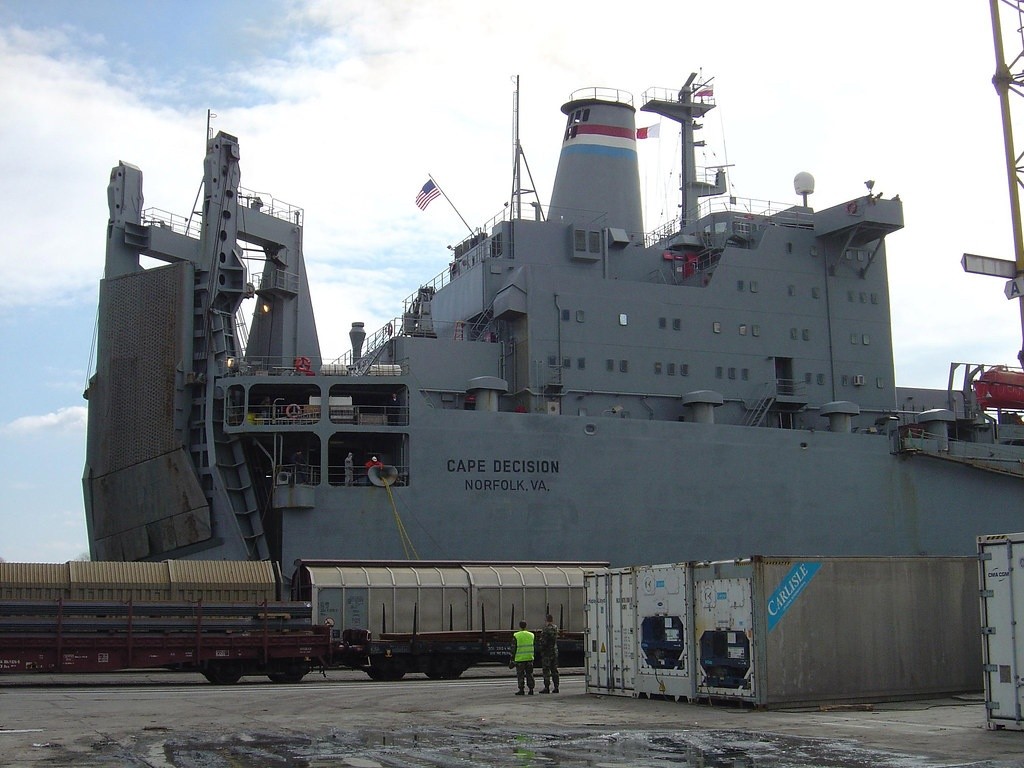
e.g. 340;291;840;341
694;84;713;96
416;179;441;211
636;123;659;139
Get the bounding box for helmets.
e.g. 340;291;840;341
372;457;377;462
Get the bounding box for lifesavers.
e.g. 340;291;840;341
847;202;858;214
286;404;300;418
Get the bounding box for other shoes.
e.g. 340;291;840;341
528;690;533;695
552;689;559;693
539;688;550;694
515;691;524;695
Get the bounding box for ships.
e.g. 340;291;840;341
74;59;1023;562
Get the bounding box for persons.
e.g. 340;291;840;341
366;456;382;486
509;621;536;695
345;453;354;486
538;615;560;693
292;449;309;485
389;393;401;425
